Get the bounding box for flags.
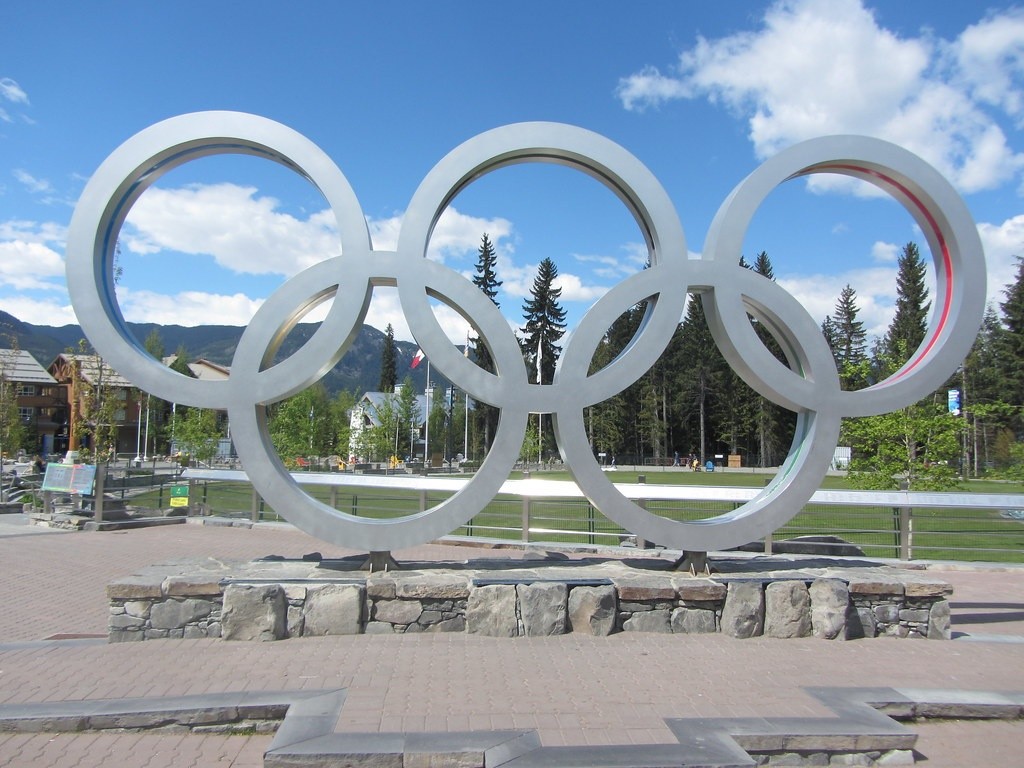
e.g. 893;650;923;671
411;348;425;369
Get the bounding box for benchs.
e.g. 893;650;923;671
354;464;372;473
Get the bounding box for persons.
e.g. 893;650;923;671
610;452;617;469
688;453;693;469
139;452;144;463
673;451;680;467
